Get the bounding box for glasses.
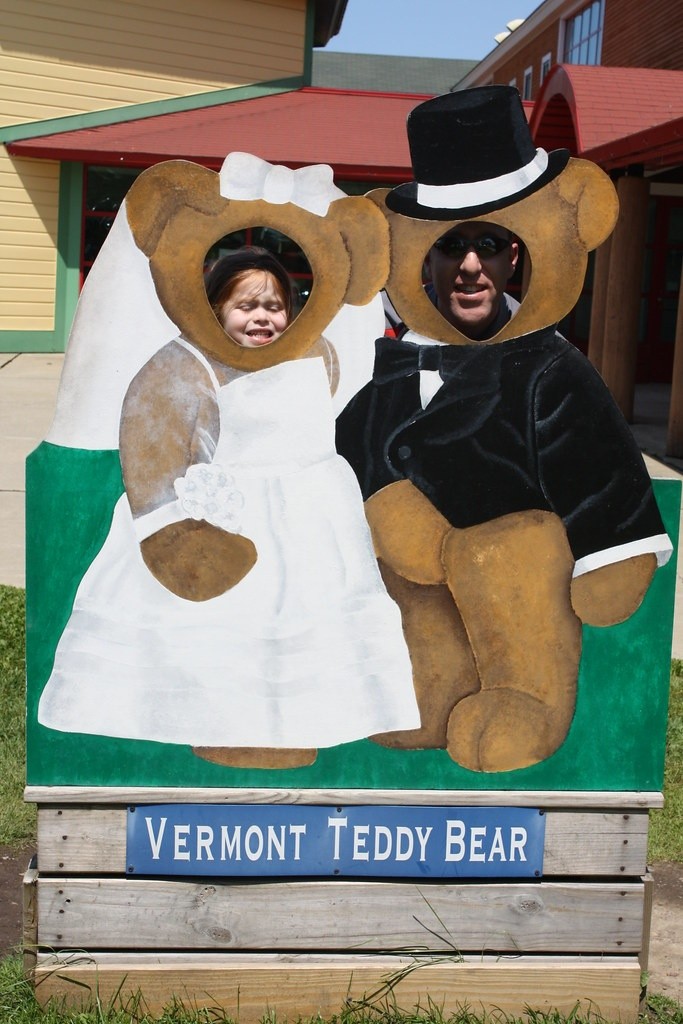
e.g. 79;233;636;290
432;231;513;259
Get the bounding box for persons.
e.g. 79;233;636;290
205;246;291;347
421;222;523;343
